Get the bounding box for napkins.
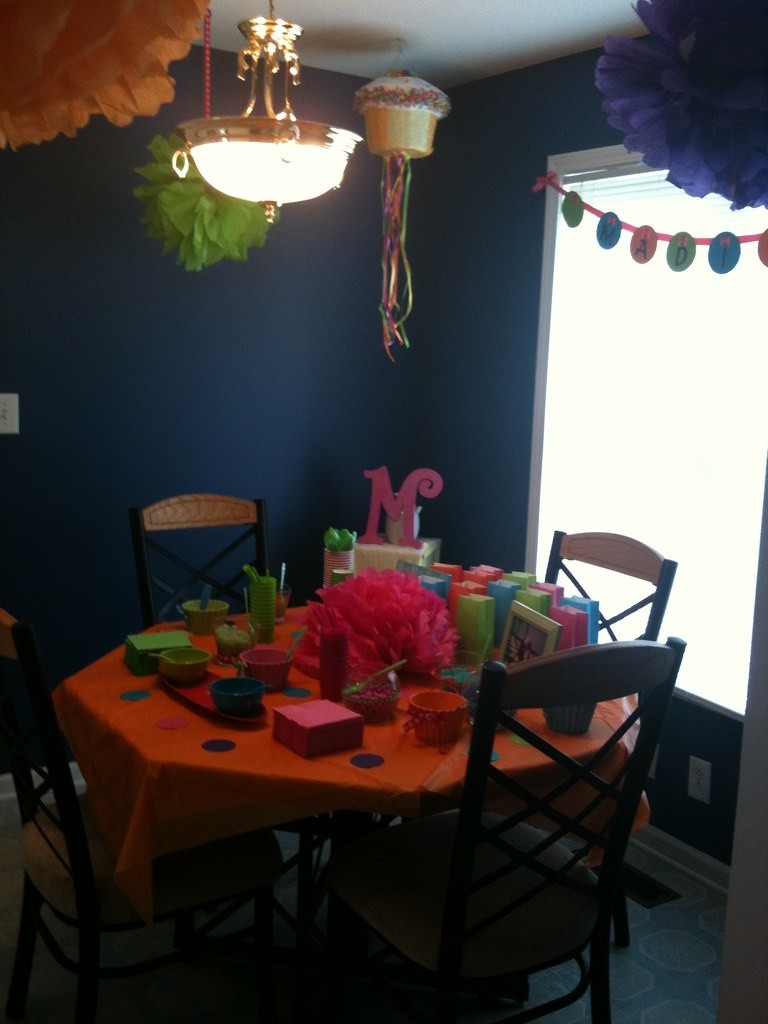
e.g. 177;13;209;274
273;699;365;760
125;630;194;676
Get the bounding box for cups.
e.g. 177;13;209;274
275;584;291;624
408;690;468;743
441;650;487;694
323;548;355;589
543;702;598;734
250;576;276;643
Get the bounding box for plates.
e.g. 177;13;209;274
158;666;266;723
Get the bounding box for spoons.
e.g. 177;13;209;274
243;587;256;648
342;659;407;695
324;527;357;551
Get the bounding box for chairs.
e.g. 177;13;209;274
129;493;336;966
321;638;686;1024
418;530;678;949
0;607;284;1024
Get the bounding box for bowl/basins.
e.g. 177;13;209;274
210;677;264;717
343;693;401;722
241;649;292;691
161;649;211;682
183;600;228;635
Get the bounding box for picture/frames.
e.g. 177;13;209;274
496;600;563;669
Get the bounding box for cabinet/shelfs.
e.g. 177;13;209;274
355;535;442;577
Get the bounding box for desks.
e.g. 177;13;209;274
61;605;629;1024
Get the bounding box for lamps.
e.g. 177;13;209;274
168;0;364;223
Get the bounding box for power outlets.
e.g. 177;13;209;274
687;755;715;804
0;393;19;435
